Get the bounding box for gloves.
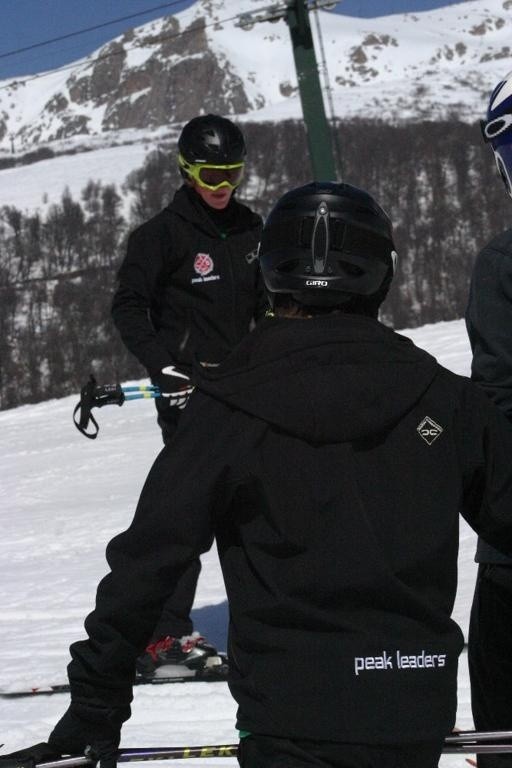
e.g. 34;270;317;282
49;703;124;767
157;363;195;409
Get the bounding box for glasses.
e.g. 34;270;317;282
178;154;246;192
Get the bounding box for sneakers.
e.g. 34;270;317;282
137;630;226;680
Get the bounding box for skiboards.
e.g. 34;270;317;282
2;672;228;701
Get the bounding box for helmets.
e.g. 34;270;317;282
177;113;249;181
258;180;399;309
485;70;512;192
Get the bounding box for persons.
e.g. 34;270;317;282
110;112;269;676
466;68;512;768
44;173;509;768
